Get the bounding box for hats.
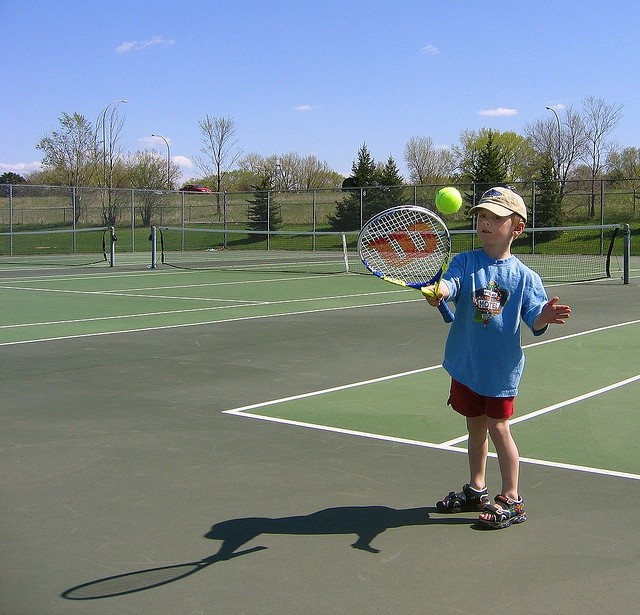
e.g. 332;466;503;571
468;187;527;224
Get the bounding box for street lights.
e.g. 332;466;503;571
545;105;560;180
151;133;170;190
103;100;128;188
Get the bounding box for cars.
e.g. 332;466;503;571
179;184;212;194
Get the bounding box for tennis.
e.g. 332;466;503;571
434;186;463;214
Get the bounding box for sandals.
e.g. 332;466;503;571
474;494;526;529
436;483;491;513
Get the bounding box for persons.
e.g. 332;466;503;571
421;186;572;530
